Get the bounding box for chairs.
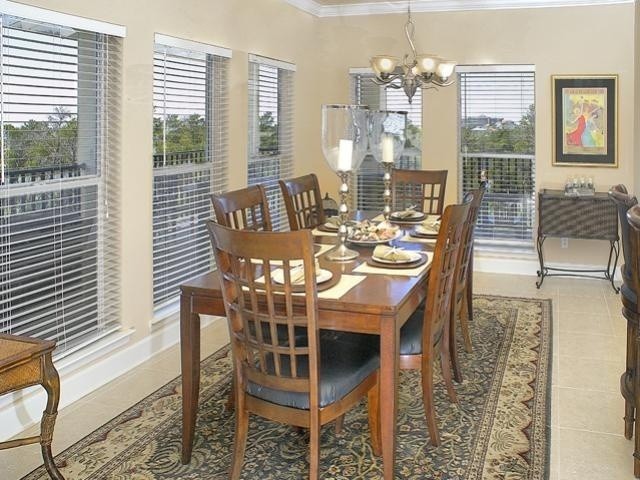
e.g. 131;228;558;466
609;184;639;266
206;166;487;480
617;203;640;479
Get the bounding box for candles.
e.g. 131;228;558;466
380;136;395;163
336;138;355;171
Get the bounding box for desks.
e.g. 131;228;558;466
534;187;624;294
0;330;67;480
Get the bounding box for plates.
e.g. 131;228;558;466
414;227;439;236
275;268;333;286
326;222;354;231
391;211;424;219
372;252;421;265
347;230;401;248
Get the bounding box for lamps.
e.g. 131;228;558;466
365;1;460;108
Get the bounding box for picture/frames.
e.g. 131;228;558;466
549;73;622;171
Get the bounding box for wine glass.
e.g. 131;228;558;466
367;110;407;221
319;103;370;262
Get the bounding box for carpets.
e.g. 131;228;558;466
17;290;555;480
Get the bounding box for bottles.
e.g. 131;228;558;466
565;178;594;190
